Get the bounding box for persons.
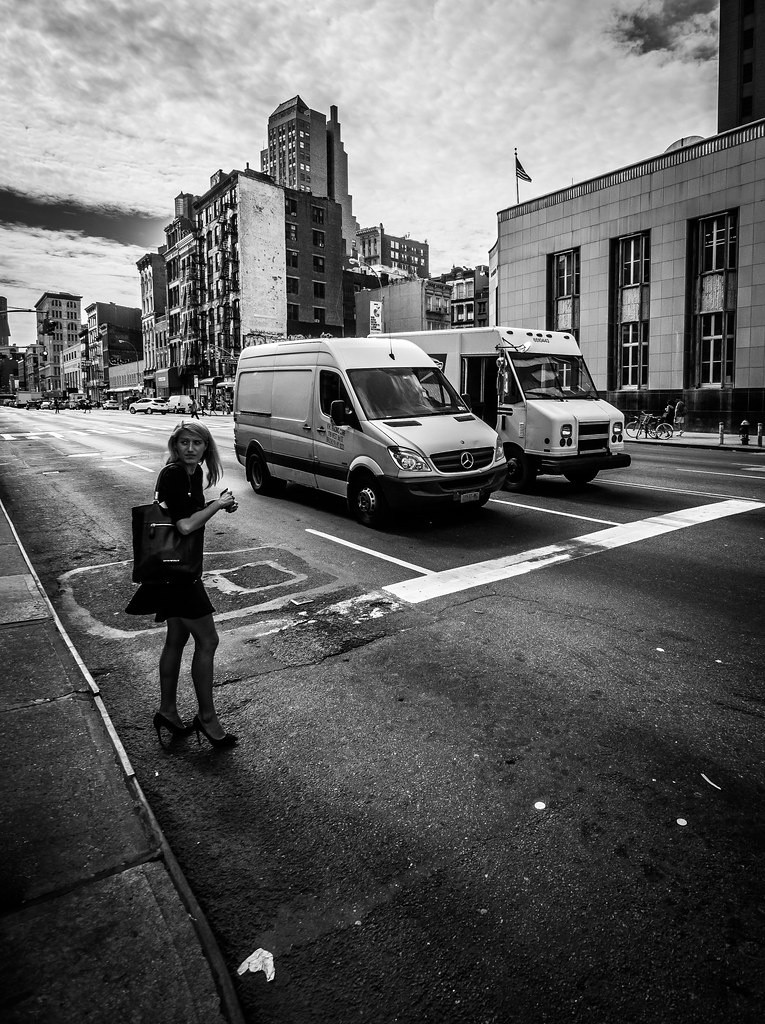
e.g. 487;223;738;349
673;396;685;437
665;400;675;437
54;398;60;414
83;397;92;413
522;366;563;396
188;395;231;420
153;419;238;751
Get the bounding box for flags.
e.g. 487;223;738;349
516;159;531;182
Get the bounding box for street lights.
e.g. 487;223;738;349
349;258;384;333
210;344;237;361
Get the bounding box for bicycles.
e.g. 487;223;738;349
625;410;674;440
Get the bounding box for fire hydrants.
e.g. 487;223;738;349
739;420;750;445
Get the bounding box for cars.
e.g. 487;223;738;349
129;398;170;414
103;399;119;409
41;401;65;409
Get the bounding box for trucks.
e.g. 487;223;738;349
168;395;194;414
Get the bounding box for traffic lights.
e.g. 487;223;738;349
119;339;140;385
49;319;57;335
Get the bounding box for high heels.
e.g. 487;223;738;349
152;712;194;742
192;714;239;747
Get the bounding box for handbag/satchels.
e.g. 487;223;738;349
132;461;203;584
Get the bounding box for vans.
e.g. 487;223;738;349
367;326;631;491
233;337;511;526
